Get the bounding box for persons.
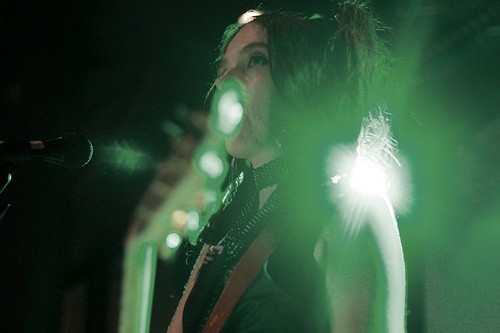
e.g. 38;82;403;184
155;0;408;333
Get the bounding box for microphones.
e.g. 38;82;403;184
0;134;93;168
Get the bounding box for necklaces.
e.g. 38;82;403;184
220;153;294;269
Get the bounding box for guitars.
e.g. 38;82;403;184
116;70;244;333
